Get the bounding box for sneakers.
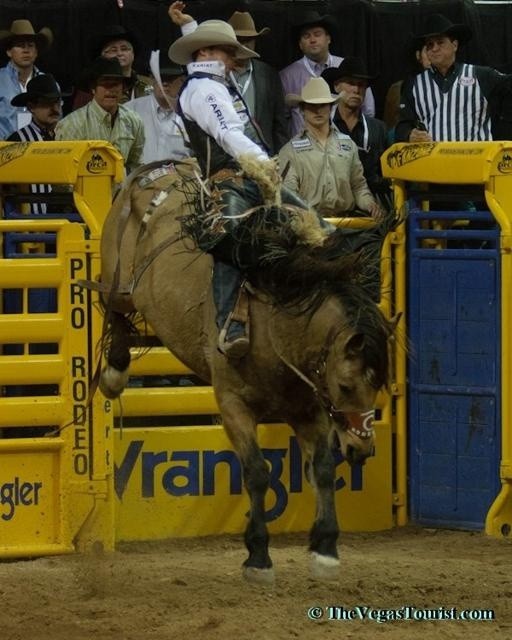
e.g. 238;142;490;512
223;333;249;355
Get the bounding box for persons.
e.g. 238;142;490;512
1;20;65;141
392;15;512;250
225;11;281;158
382;39;432;130
54;53;146;175
273;76;390;227
318;55;395;215
86;24;151;102
4;72;73;217
120;43;194;164
167;1;381;362
278;9;376;139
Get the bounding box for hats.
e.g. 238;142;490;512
413;15;471;43
168;11;271;65
285;58;374;105
11;57;130;107
290;11;337;34
0;20;42;43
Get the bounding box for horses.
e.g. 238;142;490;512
97;159;417;586
